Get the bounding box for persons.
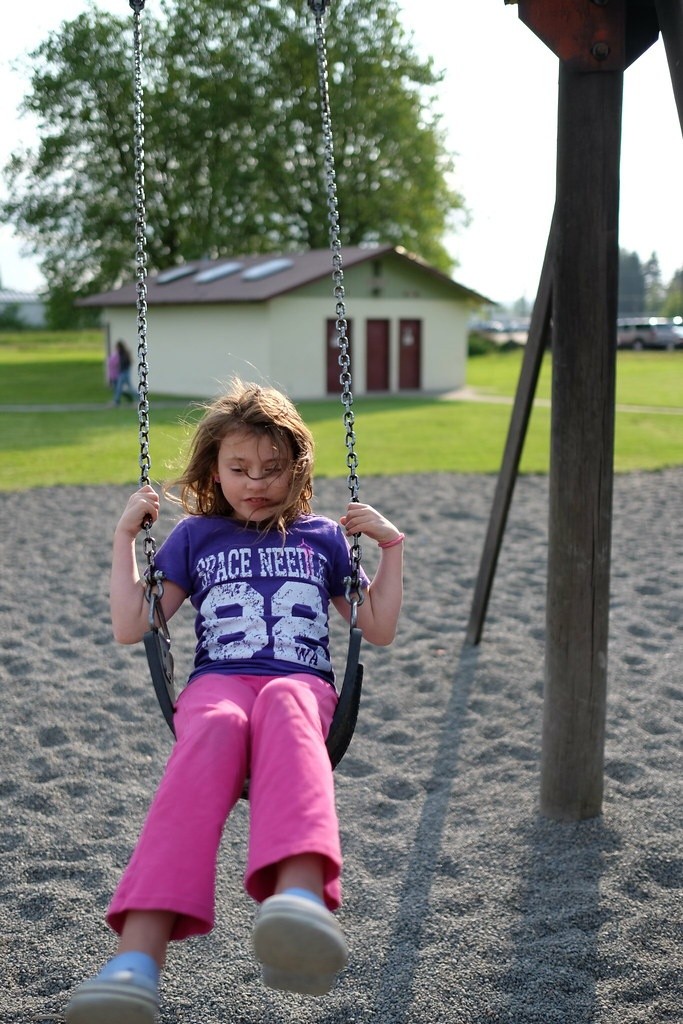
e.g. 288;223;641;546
112;342;134;405
69;385;404;1024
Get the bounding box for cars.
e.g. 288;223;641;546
472;313;683;354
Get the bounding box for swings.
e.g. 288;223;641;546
128;0;363;801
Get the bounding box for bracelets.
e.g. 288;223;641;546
378;533;405;548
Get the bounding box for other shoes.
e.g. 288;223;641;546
251;888;348;996
62;951;161;1024
